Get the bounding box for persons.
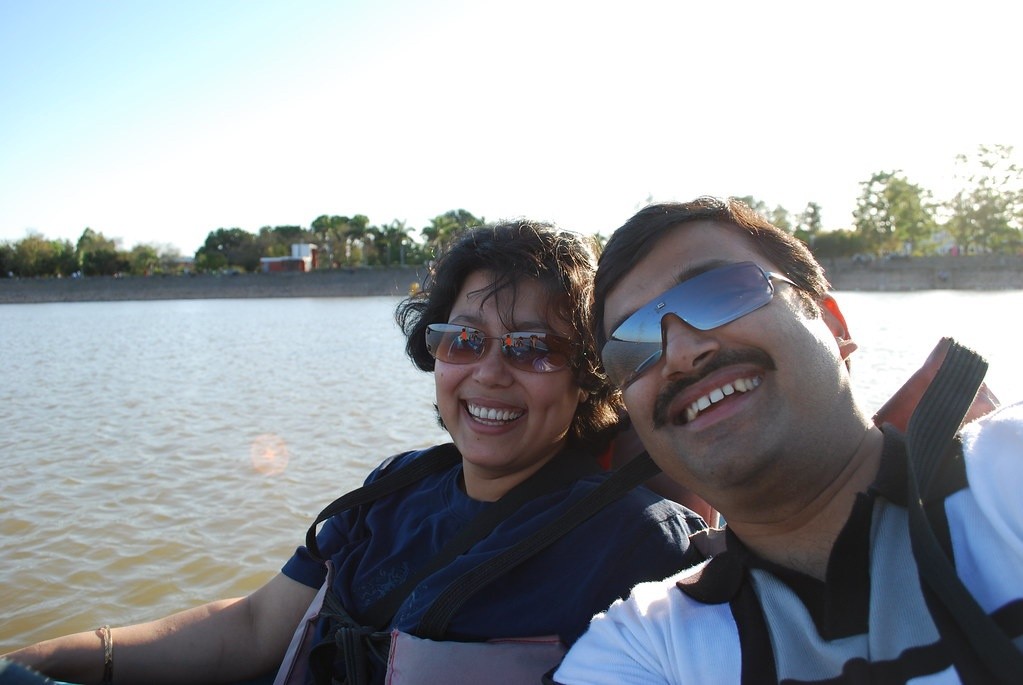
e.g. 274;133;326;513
0;222;709;685
541;196;1023;685
460;327;469;346
505;333;511;348
514;336;523;348
472;331;479;339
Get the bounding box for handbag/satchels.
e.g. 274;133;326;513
470;337;474;342
515;342;519;348
458;336;463;342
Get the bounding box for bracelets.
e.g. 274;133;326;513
98;624;112;685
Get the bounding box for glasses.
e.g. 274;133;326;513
600;262;804;392
424;321;582;373
